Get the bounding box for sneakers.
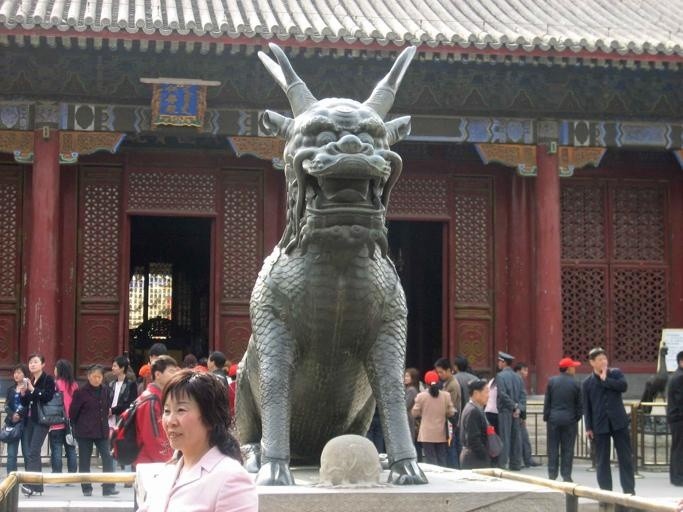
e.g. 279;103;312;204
103;488;119;495
525;460;543;467
21;485;42;496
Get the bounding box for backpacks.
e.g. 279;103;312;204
109;394;158;465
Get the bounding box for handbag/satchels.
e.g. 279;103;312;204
37;390;67;425
0;418;24;442
487;424;504;458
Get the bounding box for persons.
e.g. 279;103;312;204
131;368;260;511
372;351;541;471
665;351;683;485
0;344;239;497
579;348;635;497
544;356;583;480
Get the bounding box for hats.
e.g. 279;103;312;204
560;357;581;367
425;370;439;384
498;351;514;361
